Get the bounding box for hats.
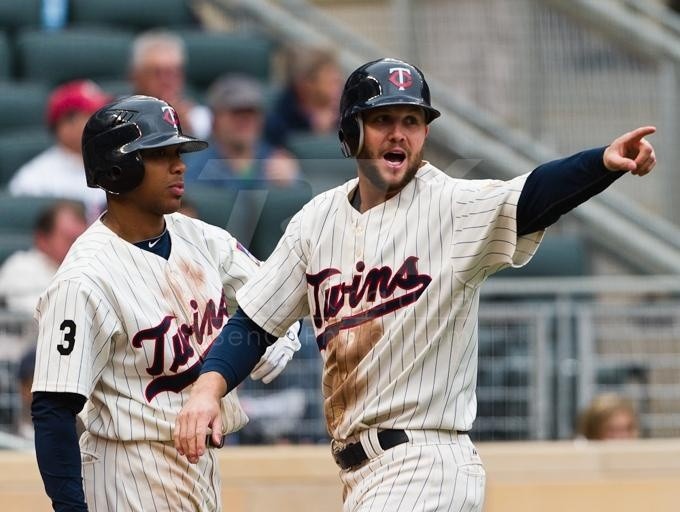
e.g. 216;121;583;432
208;73;267;112
48;81;111;122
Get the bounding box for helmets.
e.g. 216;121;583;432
336;58;441;158
82;94;209;196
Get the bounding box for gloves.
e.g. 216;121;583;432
249;316;302;386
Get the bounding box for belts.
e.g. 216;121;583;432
331;427;470;472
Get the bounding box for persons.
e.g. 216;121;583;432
173;59;657;512
0;29;351;445
29;95;308;512
581;388;643;441
